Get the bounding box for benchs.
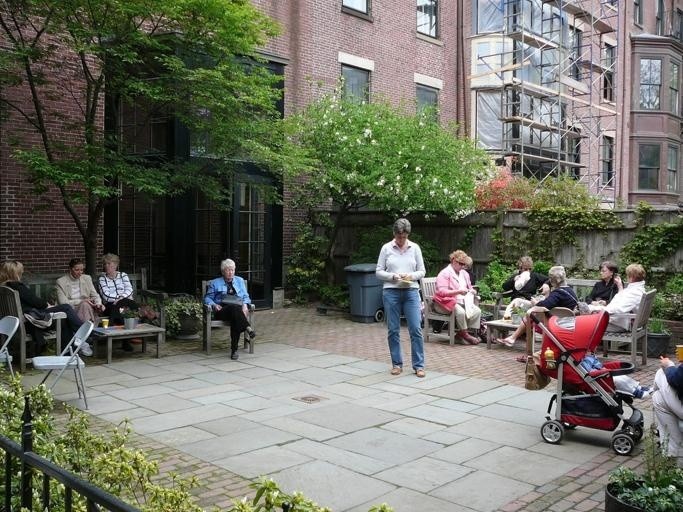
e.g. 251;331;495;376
490;279;627;350
22;265;169;352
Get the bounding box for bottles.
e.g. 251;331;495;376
545;346;554;369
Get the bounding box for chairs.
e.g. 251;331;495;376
523;307;580;367
1;286;67;372
201;279;255;355
598;287;657;366
0;316;19;379
420;275;493;345
30;320;93;411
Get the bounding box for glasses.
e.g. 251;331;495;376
522;267;534;269
450;261;469;264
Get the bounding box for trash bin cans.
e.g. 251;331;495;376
344;263;384;323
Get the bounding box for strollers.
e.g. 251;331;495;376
527;305;650;459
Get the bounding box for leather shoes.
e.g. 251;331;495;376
248;331;256;339
230;349;239;360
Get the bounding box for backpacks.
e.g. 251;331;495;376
577;301;593;315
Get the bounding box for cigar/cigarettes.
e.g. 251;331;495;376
659;355;664;360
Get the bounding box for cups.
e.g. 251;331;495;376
503;314;510;322
675;344;683;362
101;319;108;329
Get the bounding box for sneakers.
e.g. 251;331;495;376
495;337;516;348
390;367;402;376
455;332;480;346
631;385;654;400
76;341;94;357
414;368;425;378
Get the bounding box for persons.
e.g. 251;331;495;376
434;248;496;345
576;347;652;401
496;265;580;347
650;343;682;472
54;258;105;359
1;259;84;356
374;218;427;377
203;258;257;360
500;256;552;319
597;262;646;331
582;259;623;308
98;252;156;344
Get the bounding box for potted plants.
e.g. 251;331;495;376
604;410;682;511
508;302;525;325
167;299;203;341
